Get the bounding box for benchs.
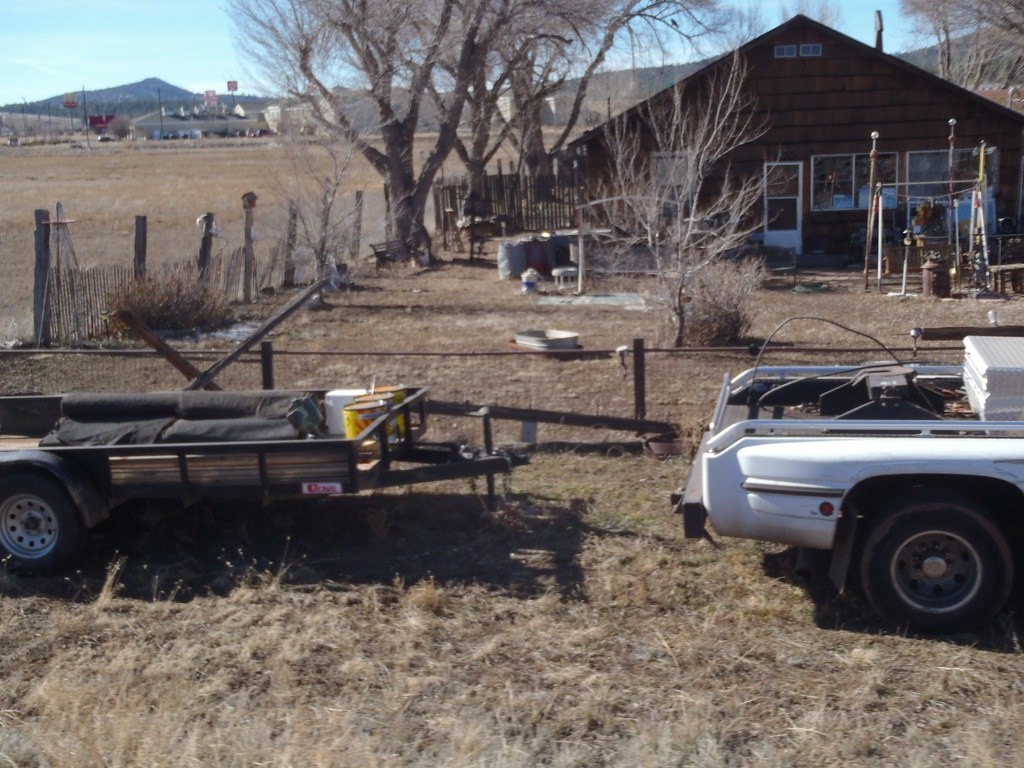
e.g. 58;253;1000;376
717;246;799;289
370;238;425;271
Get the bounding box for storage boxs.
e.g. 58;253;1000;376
517;328;580;352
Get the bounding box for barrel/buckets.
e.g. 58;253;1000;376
353;392;397;450
520;268;538;294
369;385;409;439
342;401;390;459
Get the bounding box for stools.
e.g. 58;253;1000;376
990;263;1024;294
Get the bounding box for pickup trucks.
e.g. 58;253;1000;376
674;333;1023;635
